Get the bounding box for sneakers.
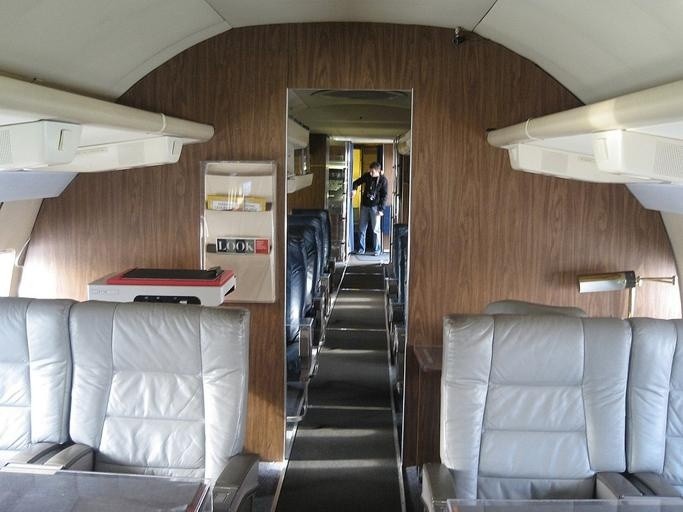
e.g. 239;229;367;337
355;250;365;255
372;253;379;256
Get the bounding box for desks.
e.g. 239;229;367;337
446;499;683;512
1;468;213;512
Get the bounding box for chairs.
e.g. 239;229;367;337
42;300;261;512
0;296;81;466
385;223;407;409
285;208;332;422
624;316;683;497
421;313;643;512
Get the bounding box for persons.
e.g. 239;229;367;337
347;162;388;256
